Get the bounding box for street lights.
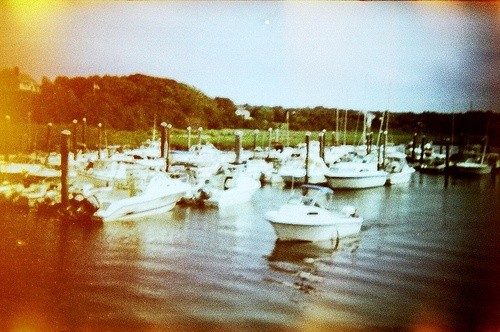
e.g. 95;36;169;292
286;111;295;147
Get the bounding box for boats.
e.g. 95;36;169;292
264;185;363;243
0;126;493;222
